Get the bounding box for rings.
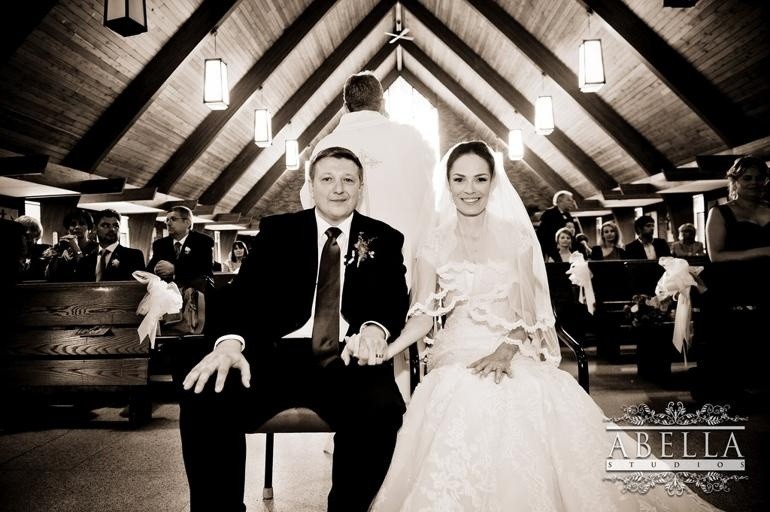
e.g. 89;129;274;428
376;354;384;358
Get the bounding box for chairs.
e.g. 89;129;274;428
155;273;244;358
407;302;591;403
221;405;337;501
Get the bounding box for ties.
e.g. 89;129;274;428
174;243;181;261
313;227;342;366
98;250;108;275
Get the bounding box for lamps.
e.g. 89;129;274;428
533;74;556;136
507;111;526;161
578;14;607;95
102;0;149;38
284;123;300;171
252;88;273;149
202;35;231;112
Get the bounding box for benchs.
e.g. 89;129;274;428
538;256;716;350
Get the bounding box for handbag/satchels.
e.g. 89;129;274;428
169;286;206;335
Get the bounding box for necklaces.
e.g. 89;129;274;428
457;210;488;267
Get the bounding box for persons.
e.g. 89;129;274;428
549;228;574;262
222;241;248;273
15;216;53;280
71;209;145;282
536;190;592;266
625;216;670;259
671;224;703;256
146;206;215;292
705;156;770;263
178;147;409;511
591;221;625;261
299;72;435;287
47;210;97;281
346;140;723;512
563;222;588;261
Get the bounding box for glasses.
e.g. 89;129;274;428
164;217;186;223
